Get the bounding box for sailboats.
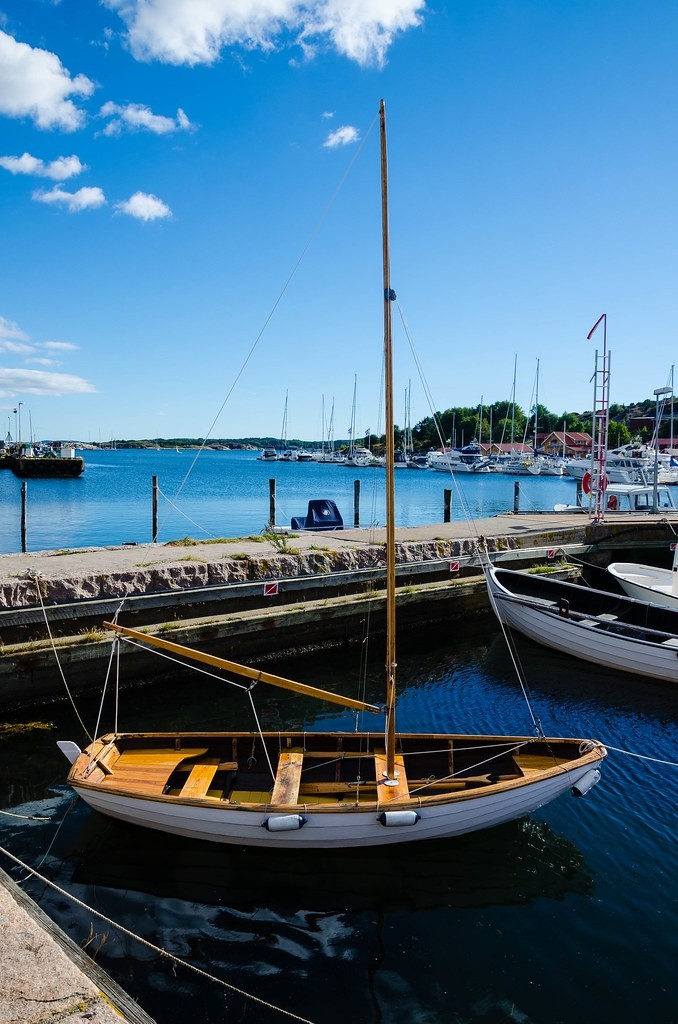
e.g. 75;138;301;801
260;354;678;483
58;100;608;852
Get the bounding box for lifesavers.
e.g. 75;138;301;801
583;468;609;497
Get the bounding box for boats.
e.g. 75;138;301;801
479;566;678;682
0;436;86;479
607;560;677;609
552;480;677;511
